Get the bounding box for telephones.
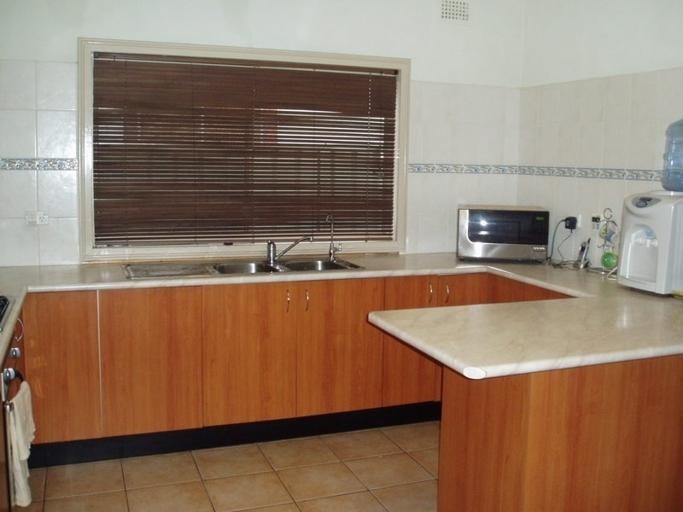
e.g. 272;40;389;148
573;238;591;269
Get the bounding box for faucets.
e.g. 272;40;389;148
326;214;342;261
266;235;313;264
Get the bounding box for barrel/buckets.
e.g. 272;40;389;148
661;119;683;191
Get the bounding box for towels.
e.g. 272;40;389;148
4;382;37;508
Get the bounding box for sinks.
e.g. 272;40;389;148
201;259;282;273
284;257;368;273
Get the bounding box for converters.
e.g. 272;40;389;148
565;216;578;229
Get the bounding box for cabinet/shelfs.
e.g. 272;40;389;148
23;288;203;466
204;277;385;449
384;272;487;428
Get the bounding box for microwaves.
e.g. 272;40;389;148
456;203;549;263
